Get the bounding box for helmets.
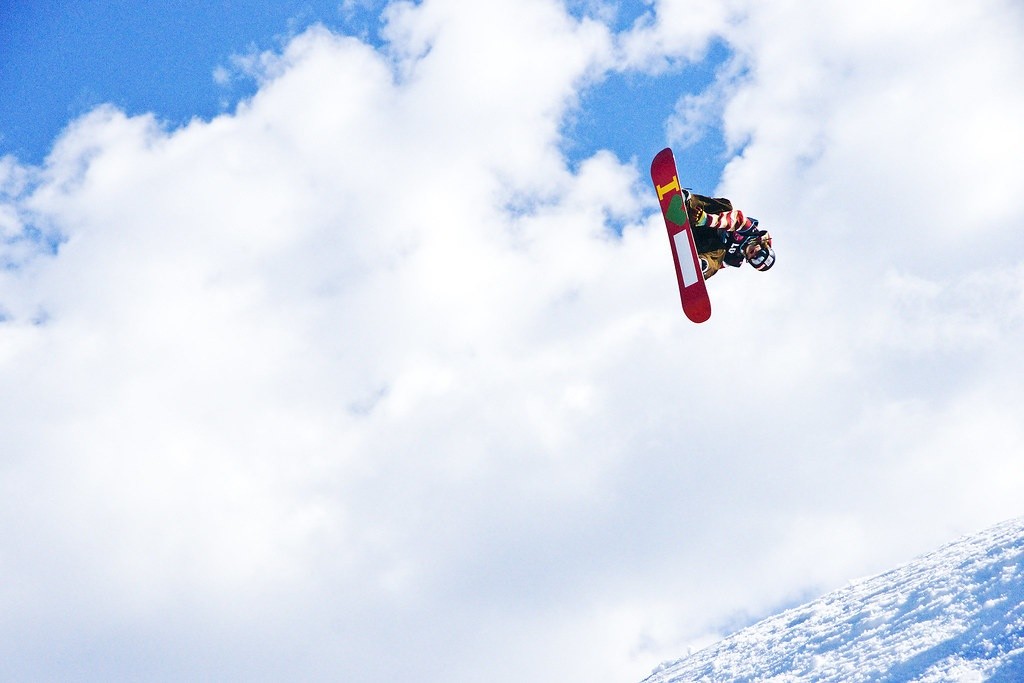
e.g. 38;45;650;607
748;246;775;271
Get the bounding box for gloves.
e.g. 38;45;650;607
691;206;703;221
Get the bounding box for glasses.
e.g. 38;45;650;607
749;248;768;268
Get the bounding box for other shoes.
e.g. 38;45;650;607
701;258;709;273
682;189;691;201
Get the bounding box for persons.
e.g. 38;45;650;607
682;188;775;281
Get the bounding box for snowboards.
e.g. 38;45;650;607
649;147;712;325
